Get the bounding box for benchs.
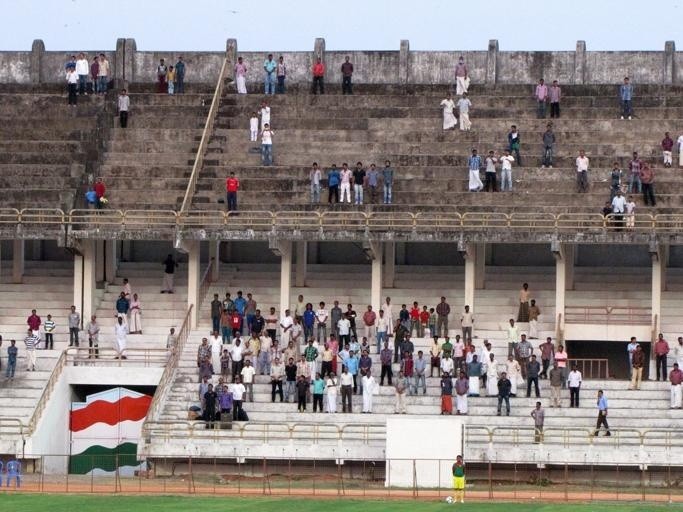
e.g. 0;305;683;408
0;78;683;472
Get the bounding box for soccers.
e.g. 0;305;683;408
446;497;453;503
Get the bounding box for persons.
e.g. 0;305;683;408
91;178;106;216
226;172;239;216
308;160;395;206
590;389;611;436
167;328;177;361
440;56;561;194
264;54;277;94
118;89;131;128
312;57;325;95
454;369;469;415
160;254;178;294
528;299;539;338
234;57;248;93
531;401;546;444
341;56;353;95
506;319;540;399
250;112;259;141
65;53;109;108
363;297;429;394
85;187;96;214
259;123;274;166
516;283;530;323
157;57;186;96
620;77;633;120
441;372;453;416
626;333;683;409
429;297;499;396
198;291;375;414
277;56;286;94
0;279;142;382
539;336;582;408
394;371;409;414
452;456;466;504
497;371;511;415
200;376;249;429
258;101;270;129
575;133;683;232
